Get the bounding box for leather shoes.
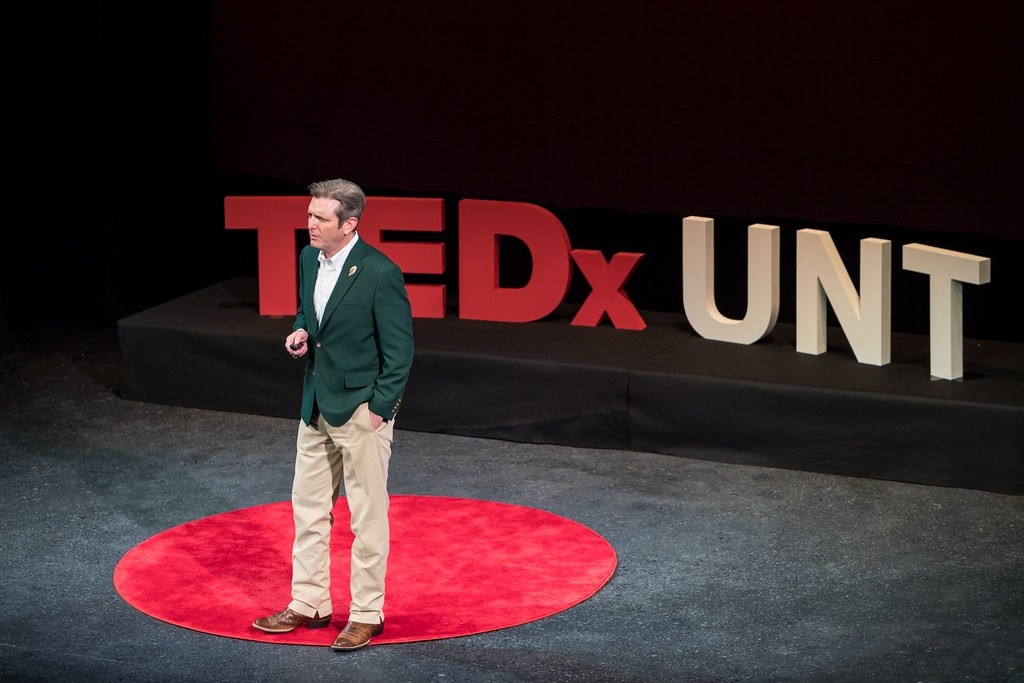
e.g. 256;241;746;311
333;618;384;650
252;608;332;633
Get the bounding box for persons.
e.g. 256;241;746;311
252;179;415;653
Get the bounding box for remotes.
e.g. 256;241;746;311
290;343;302;351
